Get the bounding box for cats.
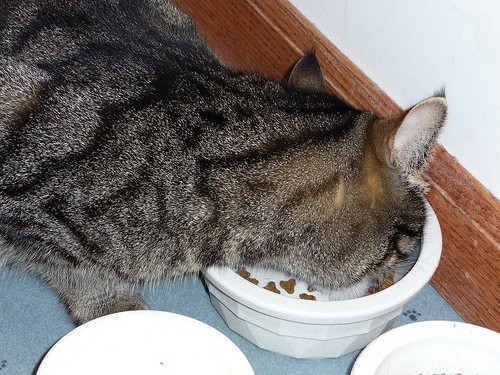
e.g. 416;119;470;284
0;0;450;331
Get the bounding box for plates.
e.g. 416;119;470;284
348;319;499;375
35;309;254;374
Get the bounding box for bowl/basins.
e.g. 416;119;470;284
202;191;442;359
